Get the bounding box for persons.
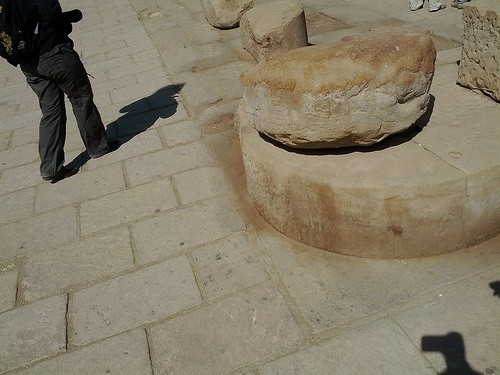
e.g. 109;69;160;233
0;0;108;183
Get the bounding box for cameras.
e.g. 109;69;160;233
61;8;83;36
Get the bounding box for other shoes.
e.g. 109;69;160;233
428;2;447;12
91;141;120;157
409;0;424;10
451;0;464;8
42;166;65;180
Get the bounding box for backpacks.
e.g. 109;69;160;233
0;0;43;67
